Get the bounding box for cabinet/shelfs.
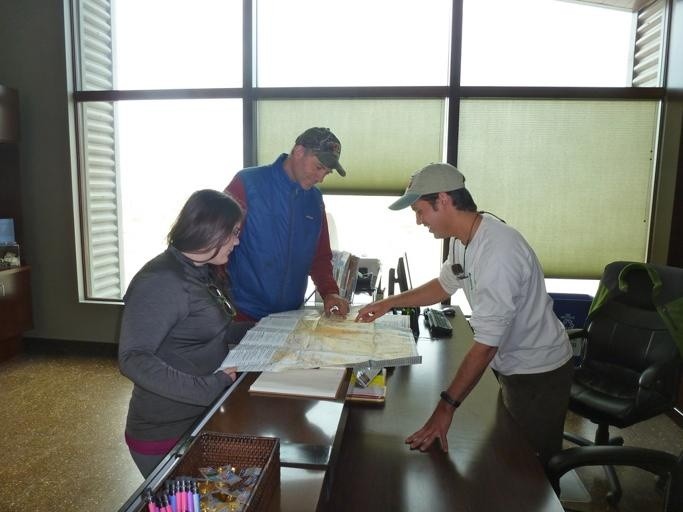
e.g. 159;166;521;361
0;81;35;363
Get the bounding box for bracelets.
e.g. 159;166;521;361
441;391;461;408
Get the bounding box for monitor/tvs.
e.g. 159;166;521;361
388;252;420;338
0;217;16;243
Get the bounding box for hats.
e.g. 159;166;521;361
387;163;465;210
294;128;346;177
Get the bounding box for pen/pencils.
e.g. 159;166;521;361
144;480;200;512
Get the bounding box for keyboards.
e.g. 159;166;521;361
424;308;452;332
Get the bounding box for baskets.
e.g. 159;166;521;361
138;431;279;511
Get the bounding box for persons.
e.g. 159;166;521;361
354;163;573;498
216;127;350;324
117;188;259;481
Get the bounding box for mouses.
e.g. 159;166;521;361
442;309;455;316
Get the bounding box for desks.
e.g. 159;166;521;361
115;301;568;512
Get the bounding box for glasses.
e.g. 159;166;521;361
207;283;237;318
451;240;468;280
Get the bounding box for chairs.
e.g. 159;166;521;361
541;261;682;504
